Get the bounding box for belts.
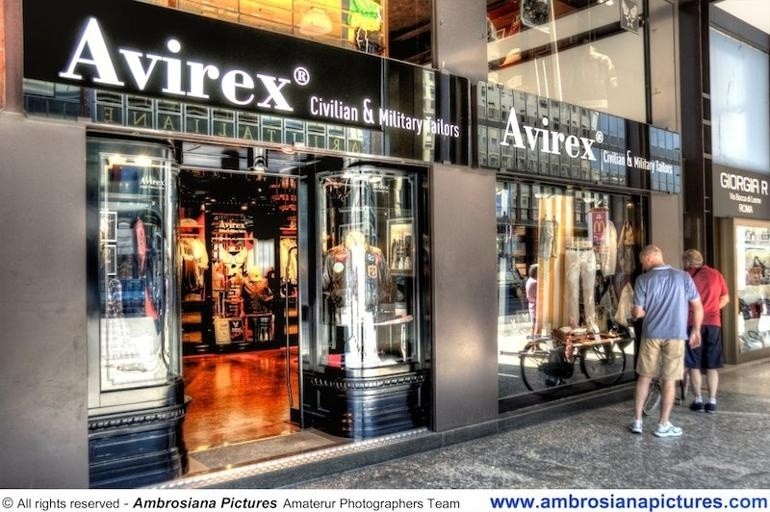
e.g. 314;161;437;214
566;246;593;251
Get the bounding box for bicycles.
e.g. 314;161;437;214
518;330;628;397
642;366;689;416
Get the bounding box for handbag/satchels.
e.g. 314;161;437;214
738;329;770;354
739;298;770;320
747;267;770;285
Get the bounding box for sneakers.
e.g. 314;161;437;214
689;400;705;412
653;423;683;438
706;402;717;414
628;418;643;433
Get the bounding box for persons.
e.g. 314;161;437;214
682;249;730;412
525;264;540;350
630;244;703;437
321;229;391;367
